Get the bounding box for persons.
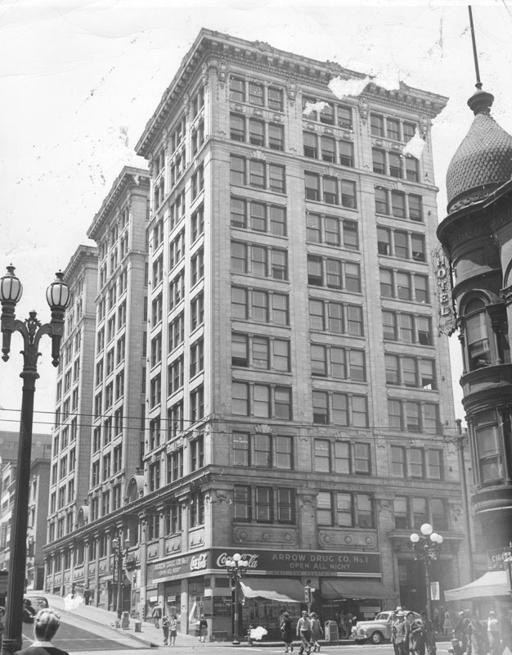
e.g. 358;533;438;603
198;615;209;644
168;615;180;647
308;611;324;653
280;612;294;653
12;608;71;655
390;597;511;655
295;611;312;655
151;603;162;629
162;616;170;644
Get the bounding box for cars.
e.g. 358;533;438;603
348;607;424;646
18;591;50;622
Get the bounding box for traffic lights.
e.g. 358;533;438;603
303;587;310;604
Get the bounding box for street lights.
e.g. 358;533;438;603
108;536;131;629
502;551;512;590
0;257;74;655
409;521;448;655
223;552;250;645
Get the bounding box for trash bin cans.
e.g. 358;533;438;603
324;620;337;641
